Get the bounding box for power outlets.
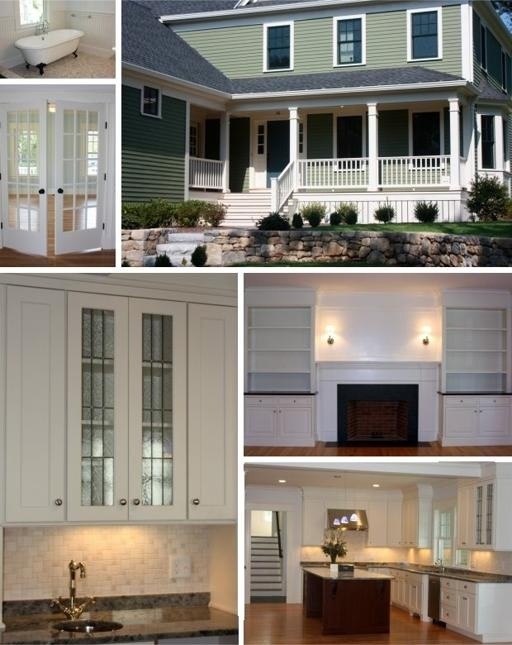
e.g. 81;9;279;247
170;556;191;579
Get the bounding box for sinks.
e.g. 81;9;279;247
418;570;440;572
52;618;124;633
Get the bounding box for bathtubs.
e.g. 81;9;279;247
13;28;86;66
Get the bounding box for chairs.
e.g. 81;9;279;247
302;567;395;636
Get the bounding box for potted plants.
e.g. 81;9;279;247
320;524;349;573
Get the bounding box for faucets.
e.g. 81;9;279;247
68;560;86;620
437;558;444;572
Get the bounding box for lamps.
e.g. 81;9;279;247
333;481;341;525
340;480;349;524
349;480;360;522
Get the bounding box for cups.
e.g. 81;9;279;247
329;564;338;573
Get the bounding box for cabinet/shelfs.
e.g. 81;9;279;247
437;290;511;447
368;486;432;549
245;285;319;447
1;273;236;528
367;562;428;623
455;478;510;552
440;578;512;645
